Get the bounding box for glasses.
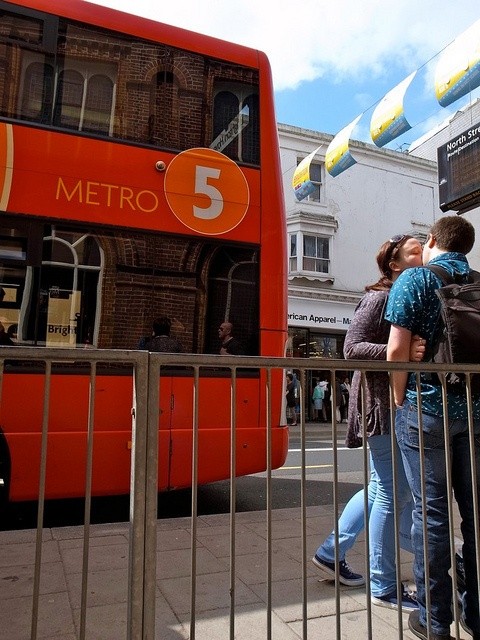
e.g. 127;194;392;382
386;234;405;261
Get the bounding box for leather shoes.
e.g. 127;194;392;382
458;611;479;639
408;610;451;639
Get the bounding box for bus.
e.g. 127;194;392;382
3;1;288;504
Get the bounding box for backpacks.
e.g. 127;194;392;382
415;265;480;393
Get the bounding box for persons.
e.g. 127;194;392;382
146;314;183;353
285;371;353;425
216;322;252;356
311;233;423;613
384;217;478;640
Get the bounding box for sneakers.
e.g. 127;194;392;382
372;582;419;614
311;552;366;587
